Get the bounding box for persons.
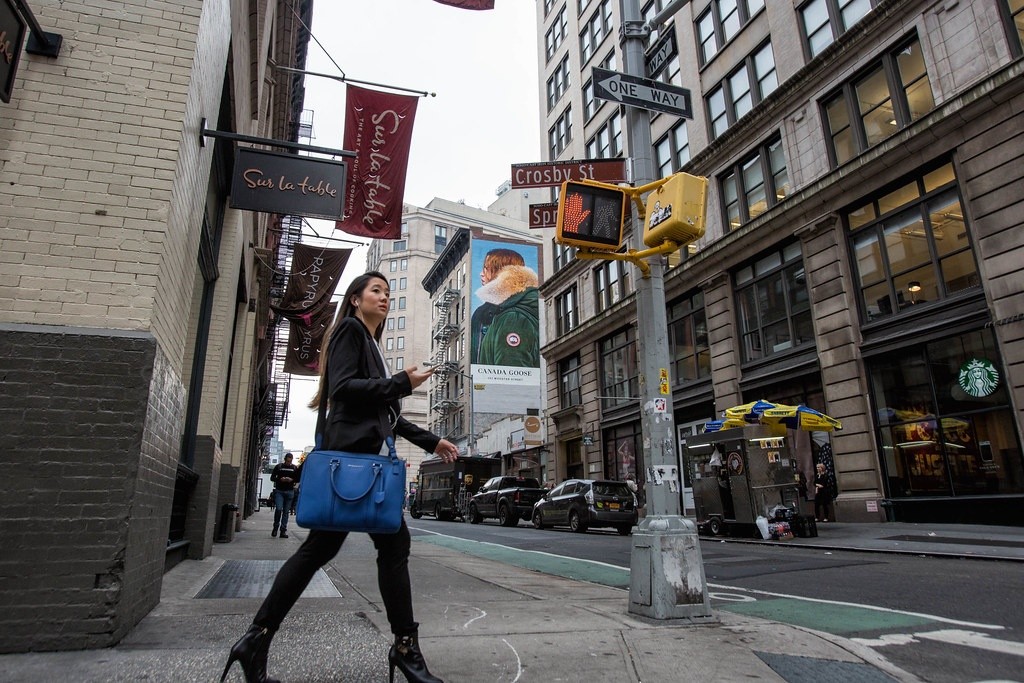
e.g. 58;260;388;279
544;481;557;489
405;492;409;510
618;441;635;478
814;464;833;521
220;271;460;683
270;453;300;538
471;248;540;367
626;473;640;526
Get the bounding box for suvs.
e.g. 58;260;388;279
531;478;639;536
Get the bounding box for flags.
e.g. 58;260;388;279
280;243;353;330
284;301;338;376
335;83;419;240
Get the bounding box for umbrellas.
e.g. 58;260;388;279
701;400;843;472
881;406;967;441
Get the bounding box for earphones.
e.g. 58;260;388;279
355;300;359;306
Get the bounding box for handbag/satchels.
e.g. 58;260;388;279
266;498;275;508
756;506;794;541
295;450;407;534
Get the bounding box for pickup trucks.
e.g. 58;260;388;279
468;475;552;528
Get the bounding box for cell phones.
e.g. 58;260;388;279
420;364;440;374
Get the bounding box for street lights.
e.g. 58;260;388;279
421;361;475;456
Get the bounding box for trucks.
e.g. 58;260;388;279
409;456;501;523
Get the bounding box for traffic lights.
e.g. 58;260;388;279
556;178;626;251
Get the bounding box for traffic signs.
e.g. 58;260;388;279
591;64;693;120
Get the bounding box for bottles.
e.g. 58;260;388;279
756;516;772;540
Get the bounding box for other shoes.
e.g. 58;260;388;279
290;514;293;515
294;513;296;515
279;534;288;538
271;532;276;537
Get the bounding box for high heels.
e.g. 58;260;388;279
220;623;281;683
388;627;444;683
